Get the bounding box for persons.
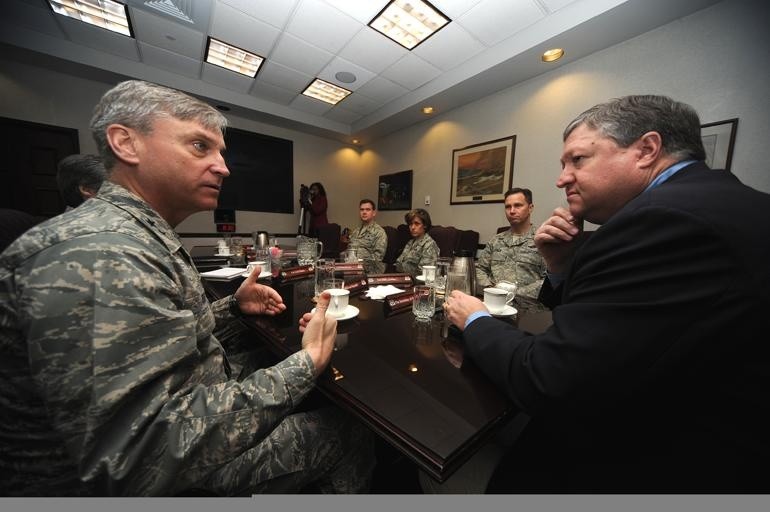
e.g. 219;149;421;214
442;94;770;493
475;188;548;299
308;183;328;238
59;155;112;214
341;200;387;262
0;80;376;495
396;209;440;271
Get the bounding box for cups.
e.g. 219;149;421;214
256;249;272;272
268;236;275;247
344;248;357;263
322;279;344;290
249;261;267;271
322;289;350;314
422;266;436;281
296;235;323;266
313;258;336;295
435;257;452;291
484;289;514;307
496;283;517;305
219;246;229;255
425;269;436;288
412;286;435;322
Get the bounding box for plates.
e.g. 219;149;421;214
242;272;272;279
215;254;235;256
482;302;518;318
311;305;361;321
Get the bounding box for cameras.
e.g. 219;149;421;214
298;184;313;207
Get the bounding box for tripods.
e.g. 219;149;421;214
297;208;318;235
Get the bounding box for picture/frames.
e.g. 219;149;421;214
700;118;739;171
449;134;517;206
377;169;414;212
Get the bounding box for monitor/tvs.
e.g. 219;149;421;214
216;127;294;214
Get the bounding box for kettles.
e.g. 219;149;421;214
444;249;477;297
256;231;270;247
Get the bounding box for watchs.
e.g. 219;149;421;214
229;295;242;317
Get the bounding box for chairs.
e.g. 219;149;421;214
496;225;510;234
383;223;480;261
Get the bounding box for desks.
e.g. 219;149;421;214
195;255;554;486
